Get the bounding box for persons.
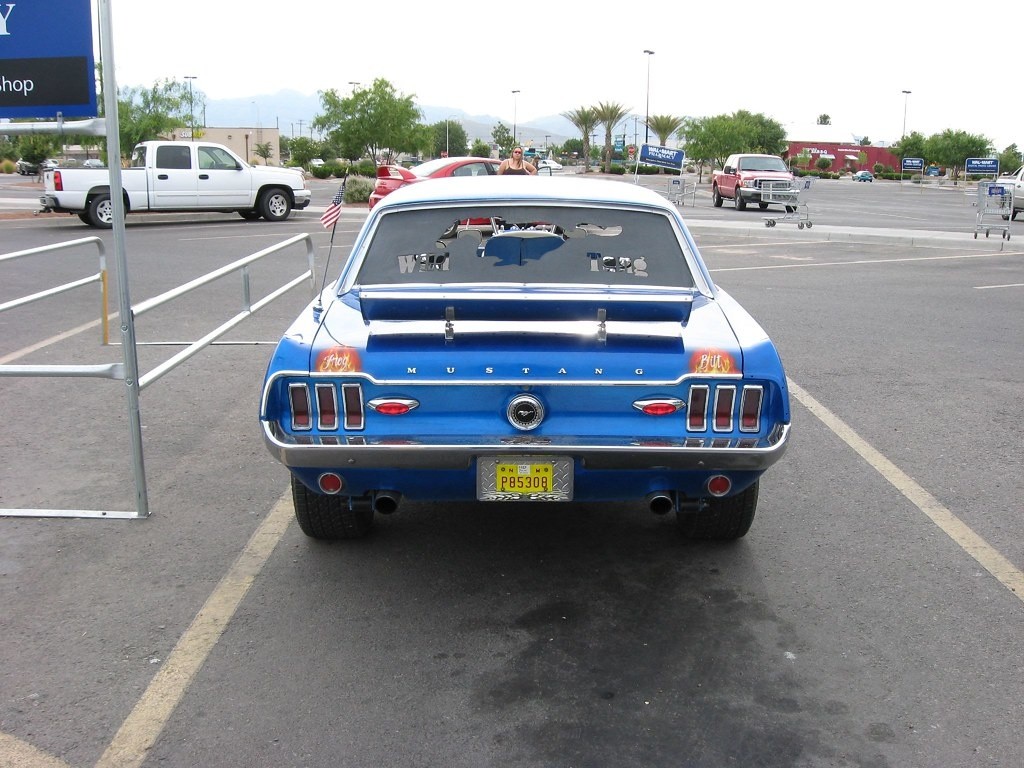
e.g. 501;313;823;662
496;146;537;175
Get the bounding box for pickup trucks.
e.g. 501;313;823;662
34;140;312;230
712;153;801;213
994;165;1024;221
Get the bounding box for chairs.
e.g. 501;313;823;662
455;167;474;175
477;168;487;175
484;230;565;265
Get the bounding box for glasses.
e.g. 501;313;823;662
514;151;521;154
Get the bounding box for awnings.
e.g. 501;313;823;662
797;154;812;159
820;154;836;160
844;155;859;160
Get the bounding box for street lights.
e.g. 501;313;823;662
512;90;520;143
349;82;360;95
902;90;911;137
184;76;198;140
644;49;655;143
545;135;551;150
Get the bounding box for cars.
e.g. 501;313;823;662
368;156;503;211
537;159;563;171
259;174;791;543
569;158;600;166
41;158;104;169
15;156;44;176
307;157;371;171
852;171;873;182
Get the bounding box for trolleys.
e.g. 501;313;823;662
760;175;820;229
974;181;1016;242
667;175;699;206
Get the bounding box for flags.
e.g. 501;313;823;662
319;183;343;229
